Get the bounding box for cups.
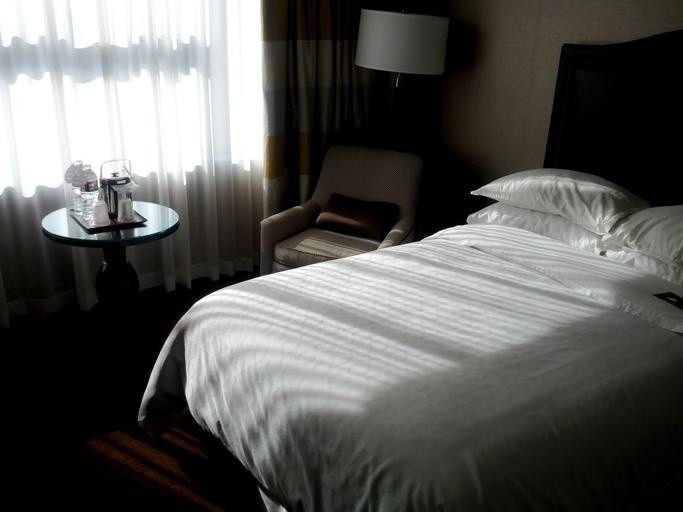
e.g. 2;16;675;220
66;186;134;227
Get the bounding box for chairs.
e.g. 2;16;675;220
258;144;427;276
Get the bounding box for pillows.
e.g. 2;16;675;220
316;191;403;244
469;166;650;235
466;203;608;259
603;205;683;270
603;243;683;288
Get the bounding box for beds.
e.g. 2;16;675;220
139;28;683;511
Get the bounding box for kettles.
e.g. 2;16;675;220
99;172;132;220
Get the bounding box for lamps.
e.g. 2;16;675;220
355;7;449;95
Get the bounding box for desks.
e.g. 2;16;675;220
41;200;177;363
